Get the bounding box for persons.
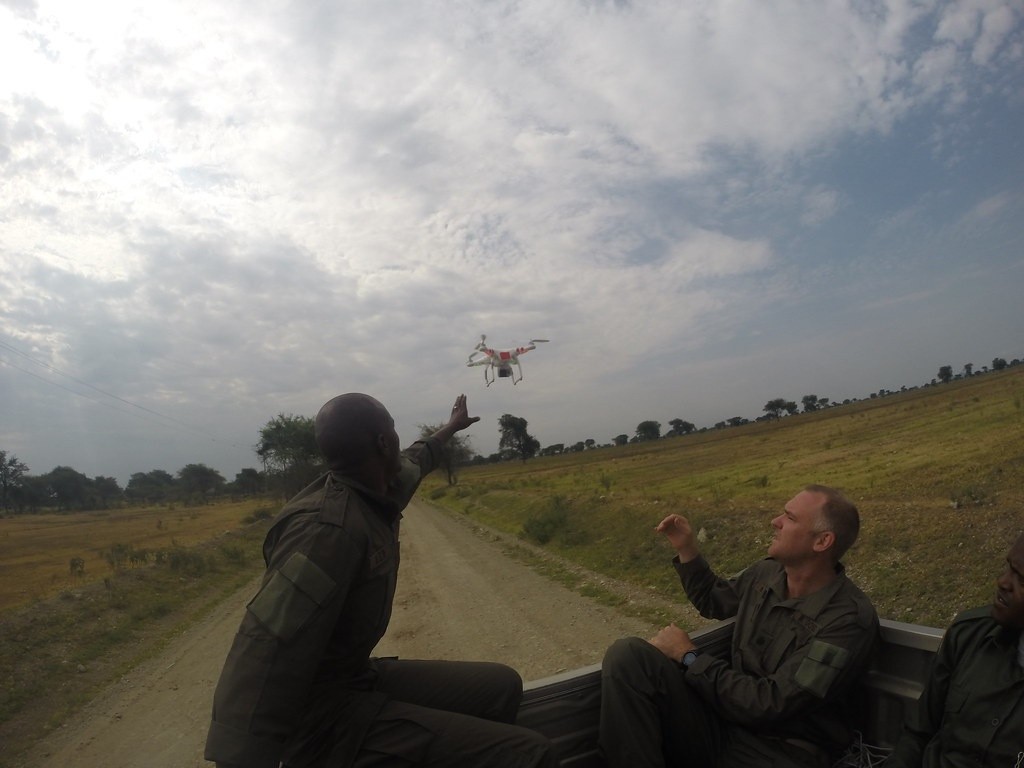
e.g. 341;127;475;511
593;484;884;768
202;391;561;768
888;532;1024;768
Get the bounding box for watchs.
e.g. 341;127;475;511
678;647;706;672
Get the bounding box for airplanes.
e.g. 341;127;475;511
467;333;550;387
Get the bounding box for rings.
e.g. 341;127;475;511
453;406;458;409
